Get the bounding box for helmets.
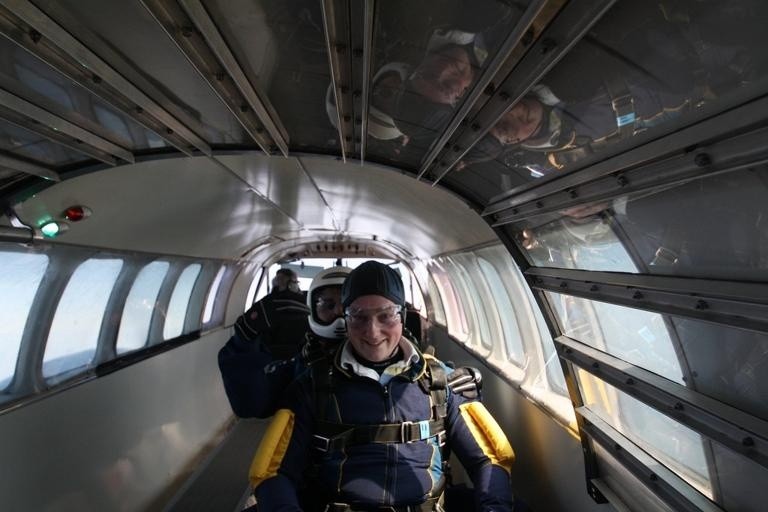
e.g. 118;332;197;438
305;265;354;339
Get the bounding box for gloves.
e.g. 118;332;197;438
232;288;309;344
447;363;484;399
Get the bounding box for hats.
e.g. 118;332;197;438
339;260;406;312
